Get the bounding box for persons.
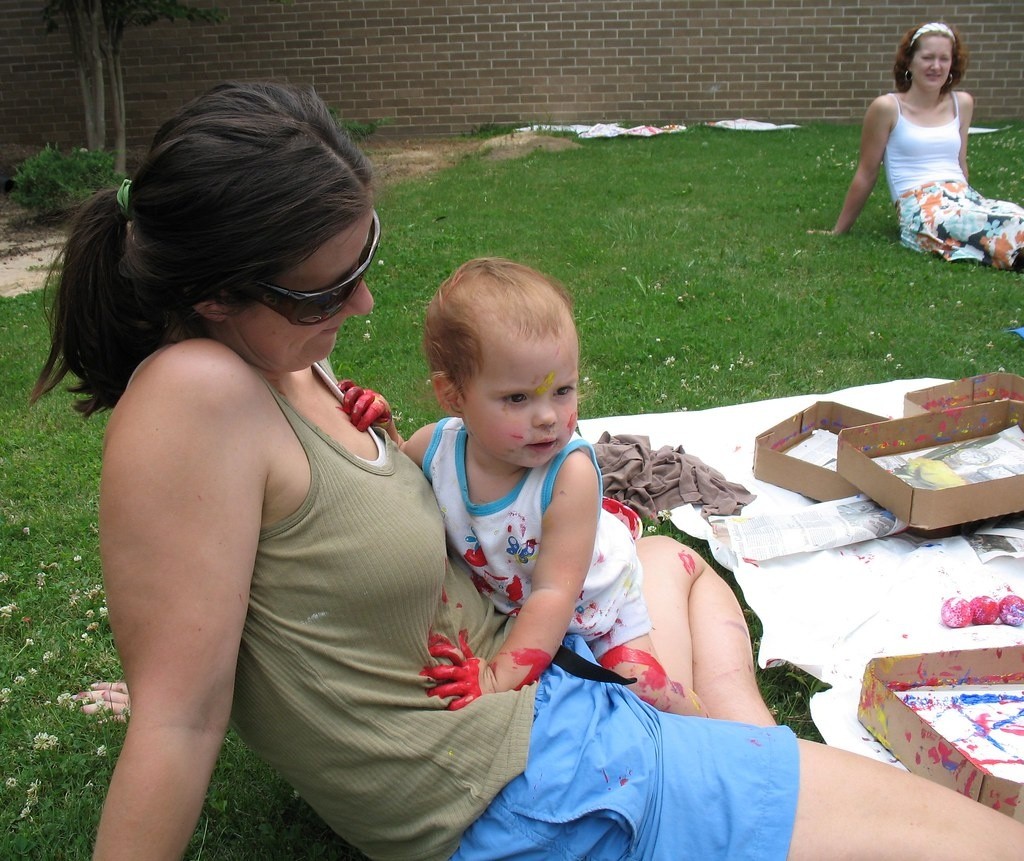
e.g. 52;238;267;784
55;82;1024;860
802;22;1024;276
337;258;716;726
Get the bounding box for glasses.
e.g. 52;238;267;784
224;209;381;326
